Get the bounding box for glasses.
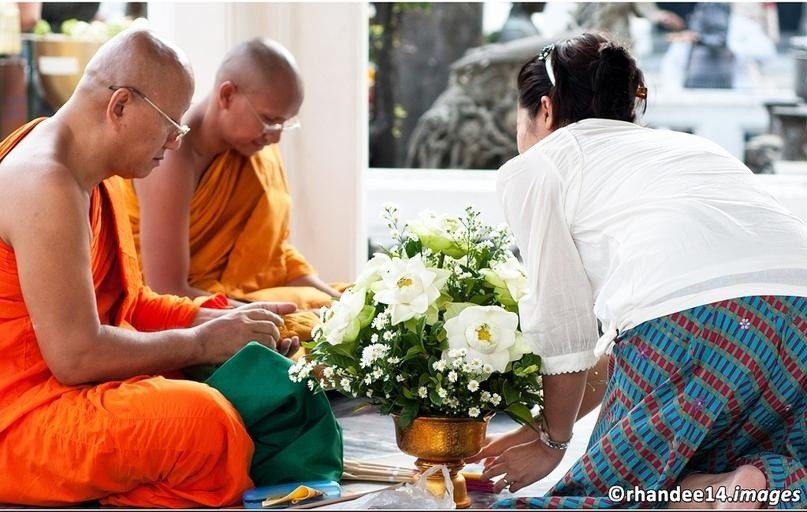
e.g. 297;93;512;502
108;85;191;140
237;84;300;133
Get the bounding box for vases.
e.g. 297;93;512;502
390;409;495;508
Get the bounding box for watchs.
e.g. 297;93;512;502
537;422;574;450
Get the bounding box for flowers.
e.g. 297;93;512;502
287;203;549;436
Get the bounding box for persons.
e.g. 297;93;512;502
18;3;109;33
462;31;806;512
0;27;309;510
121;36;355;388
559;2;806;90
